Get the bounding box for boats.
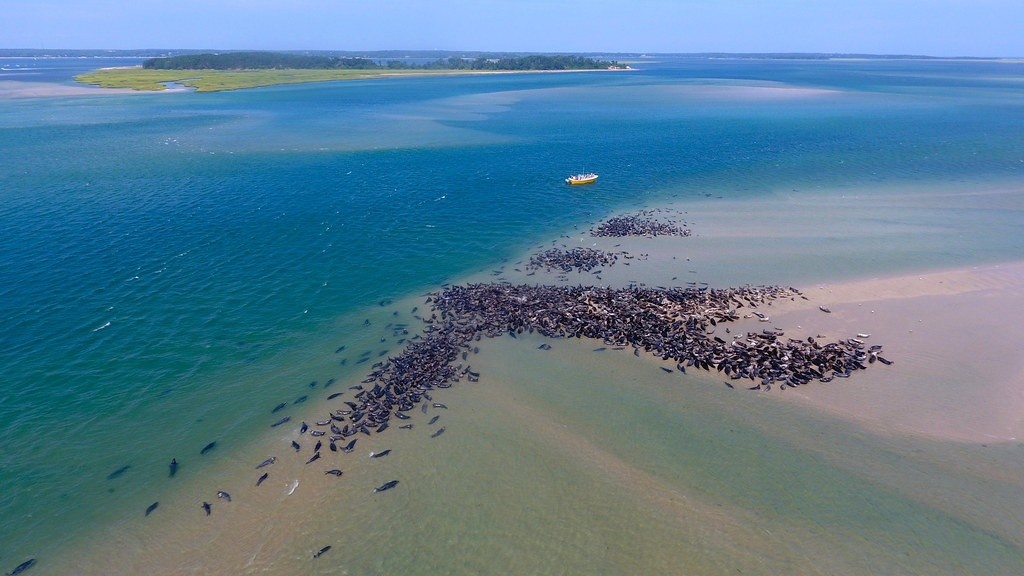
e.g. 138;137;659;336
566;172;599;184
